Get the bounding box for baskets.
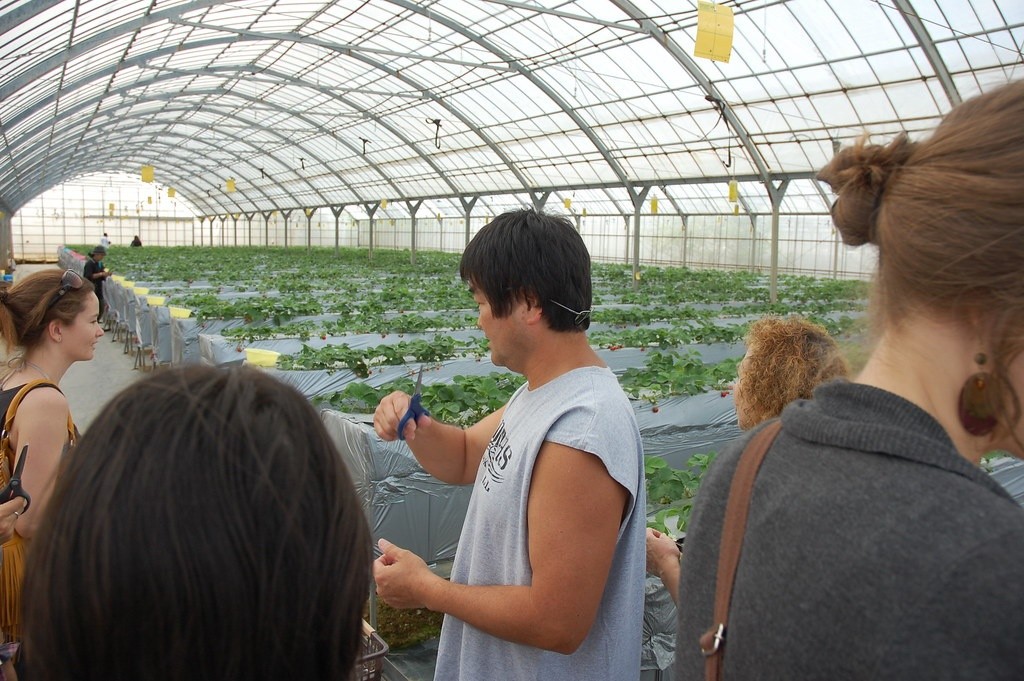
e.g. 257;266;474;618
347;616;389;681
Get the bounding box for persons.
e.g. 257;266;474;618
364;207;646;681
18;358;373;681
665;74;1023;680
0;268;105;681
0;495;25;545
129;235;142;247
646;315;855;623
101;233;111;245
83;245;113;323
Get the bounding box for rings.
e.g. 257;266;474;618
14;511;20;519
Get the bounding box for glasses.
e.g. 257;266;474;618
544;299;592;326
47;268;83;310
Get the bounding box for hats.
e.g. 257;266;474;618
92;246;105;255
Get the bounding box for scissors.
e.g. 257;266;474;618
397;364;431;441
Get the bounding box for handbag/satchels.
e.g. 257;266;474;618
0;379;77;500
